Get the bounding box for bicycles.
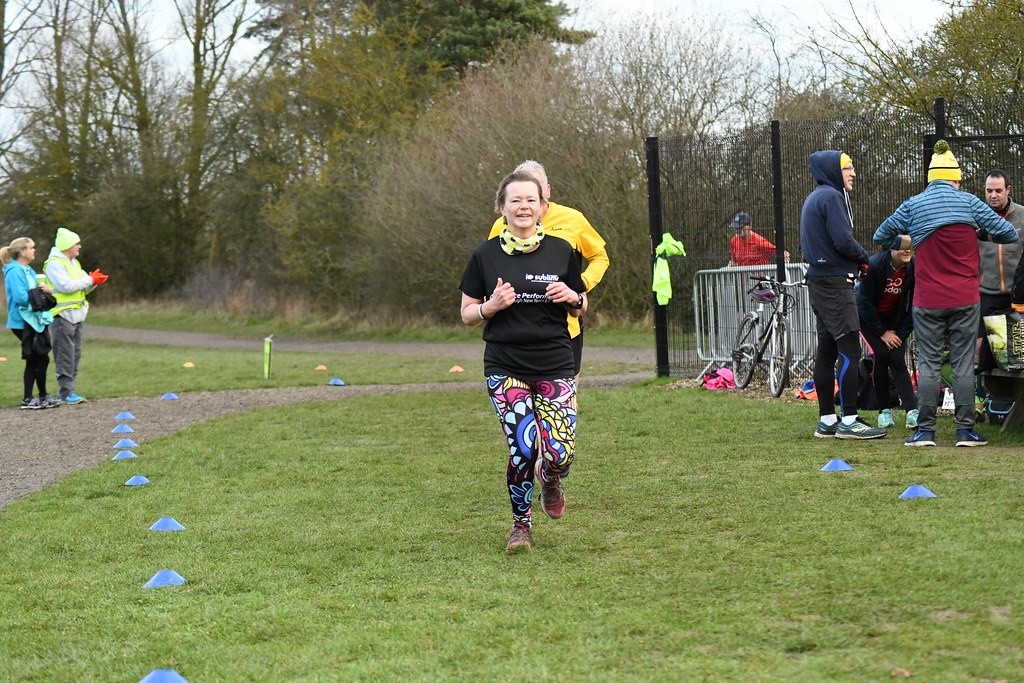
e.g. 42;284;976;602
730;275;809;398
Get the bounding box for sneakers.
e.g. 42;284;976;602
534;456;567;518
813;413;841;438
904;408;920;428
877;409;895;428
904;429;936;447
20;396;46;410
955;430;989;447
53;390;87;404
506;521;535;553
834;415;887;440
40;395;60;407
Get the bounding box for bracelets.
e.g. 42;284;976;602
479;304;487;321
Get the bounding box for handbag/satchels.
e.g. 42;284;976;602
983;311;1024;374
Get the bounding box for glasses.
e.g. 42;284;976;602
843;165;855;174
735;223;748;230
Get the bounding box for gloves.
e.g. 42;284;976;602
88;268;109;285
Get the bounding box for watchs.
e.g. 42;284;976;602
570;295;583;309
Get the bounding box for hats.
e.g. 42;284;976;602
55;227;80;251
839;152;853;170
728;212;751;229
927;140;962;183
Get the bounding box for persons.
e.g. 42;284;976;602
873;140;1018;446
858;249;918;428
489;161;609;386
44;228;108;404
724;212;790;266
0;237;62;409
800;151;887;437
973;169;1024;424
460;174;587;546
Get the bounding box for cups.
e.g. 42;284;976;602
35;273;47;286
